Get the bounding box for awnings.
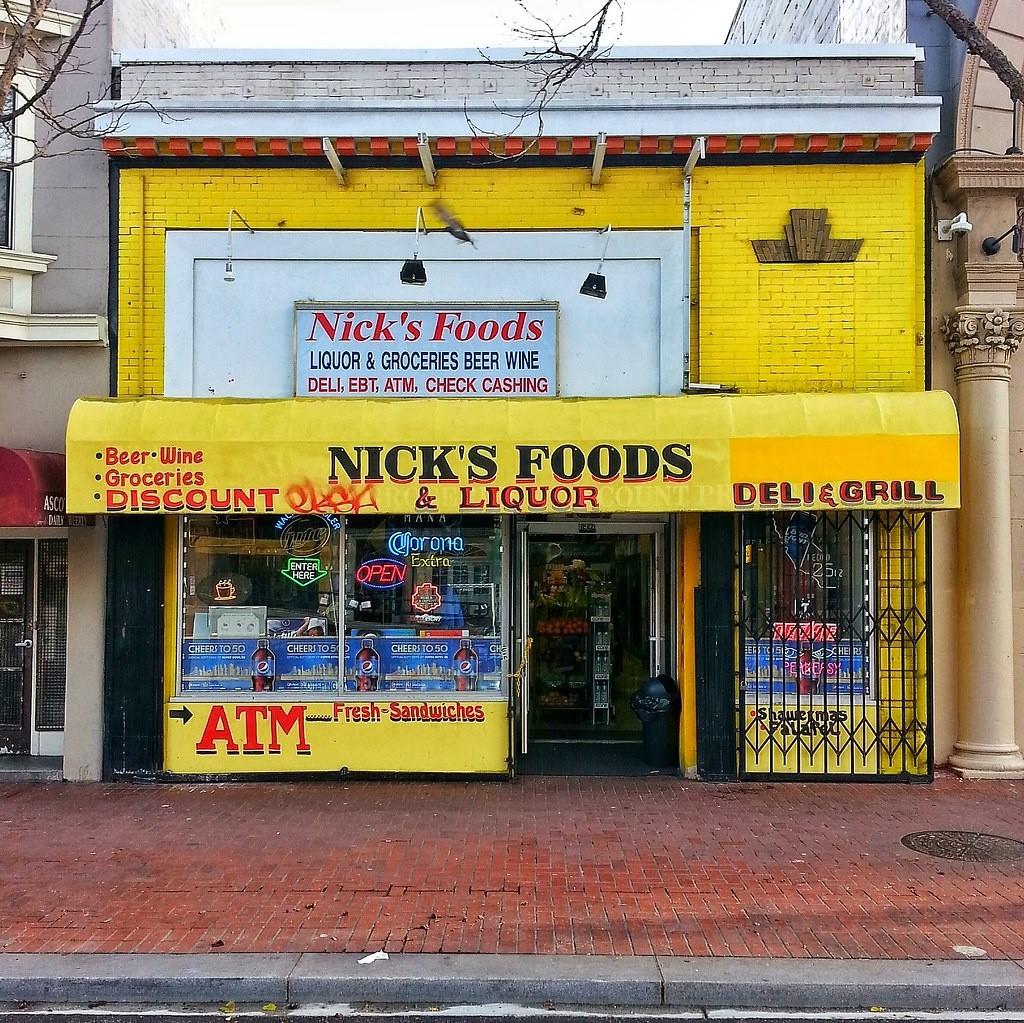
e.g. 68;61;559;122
0;446;97;527
67;390;961;513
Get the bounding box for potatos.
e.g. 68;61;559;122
539;691;568;706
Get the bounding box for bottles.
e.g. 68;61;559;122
453;638;479;692
249;639;276;692
595;605;607;703
355;638;380;692
799;642;819;694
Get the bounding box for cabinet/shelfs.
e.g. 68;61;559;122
534;587;613;727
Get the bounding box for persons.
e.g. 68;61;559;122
297;617;324;636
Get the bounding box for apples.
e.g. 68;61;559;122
535;617;588;634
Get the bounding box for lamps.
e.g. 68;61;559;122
938;212;972;241
579;222;611;299
224;208;253;281
983;225;1020;254
399;206;429;286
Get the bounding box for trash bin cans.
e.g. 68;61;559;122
638;675;681;766
